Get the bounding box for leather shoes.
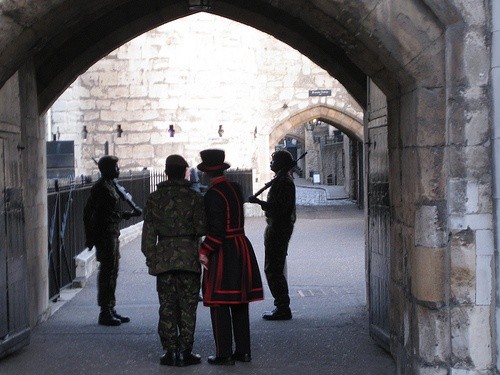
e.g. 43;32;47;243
208;356;234;366
234;352;251;362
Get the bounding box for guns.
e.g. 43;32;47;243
253;151;308;198
91;156;143;216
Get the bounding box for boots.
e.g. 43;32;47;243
263;307;292;320
99;307;129;325
178;352;201;367
160;348;177;367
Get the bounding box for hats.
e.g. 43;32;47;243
167;155;189;166
98;156;119;165
197;150;230;171
271;150;292;159
